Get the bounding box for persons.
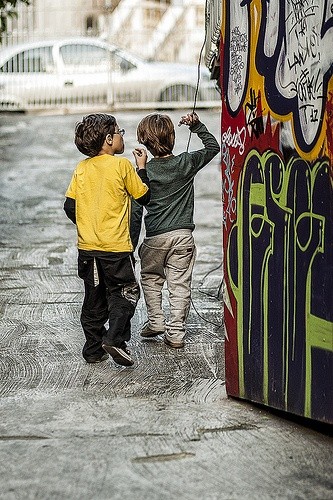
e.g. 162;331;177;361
130;112;220;348
62;113;151;367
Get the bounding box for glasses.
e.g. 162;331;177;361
111;128;126;138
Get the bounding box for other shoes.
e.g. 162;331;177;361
162;332;185;349
140;319;166;336
103;343;136;368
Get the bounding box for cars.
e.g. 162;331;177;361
0;36;221;115
